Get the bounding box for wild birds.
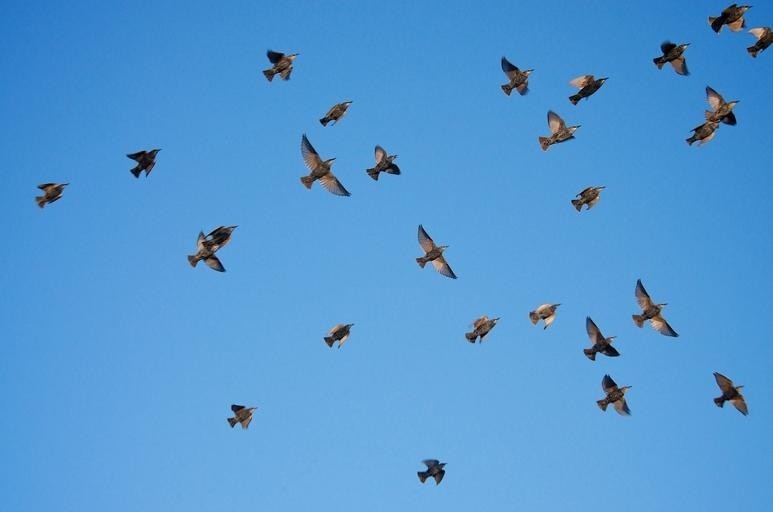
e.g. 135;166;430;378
712;371;748;416
187;225;237;273
465;278;679;361
323;323;354;348
500;58;608;151
595;373;632;416
35;183;68;208
416;459;447;485
262;51;300;81
415;223;457;279
653;3;773;149
125;149;161;179
227;404;258;429
299;100;400;197
570;185;606;212
